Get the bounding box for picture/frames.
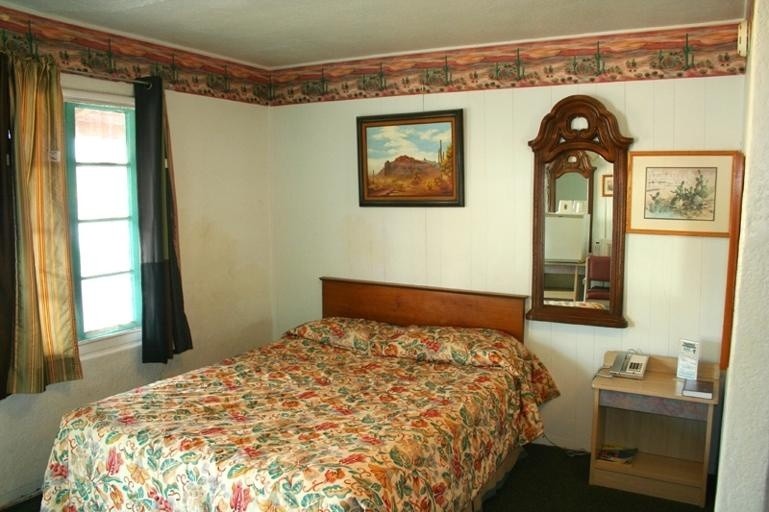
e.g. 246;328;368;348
623;148;746;238
601;173;614;199
355;108;466;206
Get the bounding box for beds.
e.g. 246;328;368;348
41;278;564;511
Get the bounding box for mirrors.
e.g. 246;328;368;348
545;150;598;216
526;95;633;329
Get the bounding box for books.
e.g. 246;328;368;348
598;442;639;468
680;379;715;400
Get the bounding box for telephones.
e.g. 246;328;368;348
608;352;649;380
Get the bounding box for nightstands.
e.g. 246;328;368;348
585;353;721;508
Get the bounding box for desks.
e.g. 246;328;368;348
544;255;586;302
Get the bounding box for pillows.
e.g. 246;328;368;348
368;327;519;368
286;314;399;350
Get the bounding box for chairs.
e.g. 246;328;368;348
583;253;613;303
